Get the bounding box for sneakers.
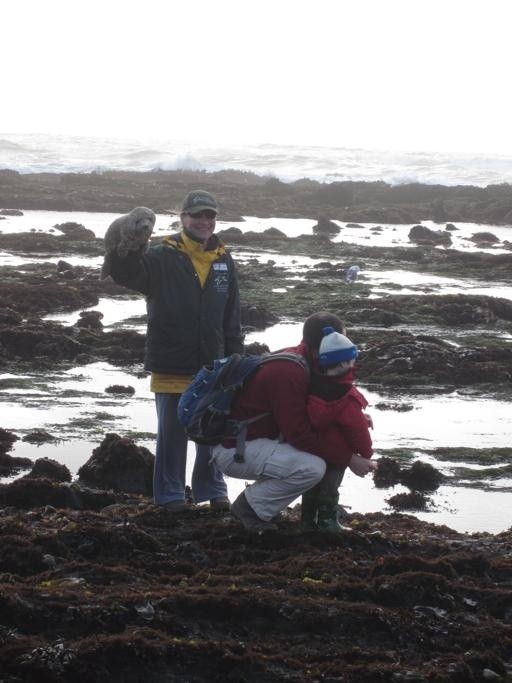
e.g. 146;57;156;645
211;497;229;513
229;491;277;533
158;499;191;514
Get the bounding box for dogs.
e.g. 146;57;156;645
100;206;156;281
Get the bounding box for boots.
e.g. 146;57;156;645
300;495;353;539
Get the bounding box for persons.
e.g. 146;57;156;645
298;326;374;532
209;311;379;533
108;189;246;512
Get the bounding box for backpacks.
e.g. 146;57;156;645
177;351;311;447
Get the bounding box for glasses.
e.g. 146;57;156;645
187;210;217;220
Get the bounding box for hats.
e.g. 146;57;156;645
318;326;358;365
179;189;219;216
